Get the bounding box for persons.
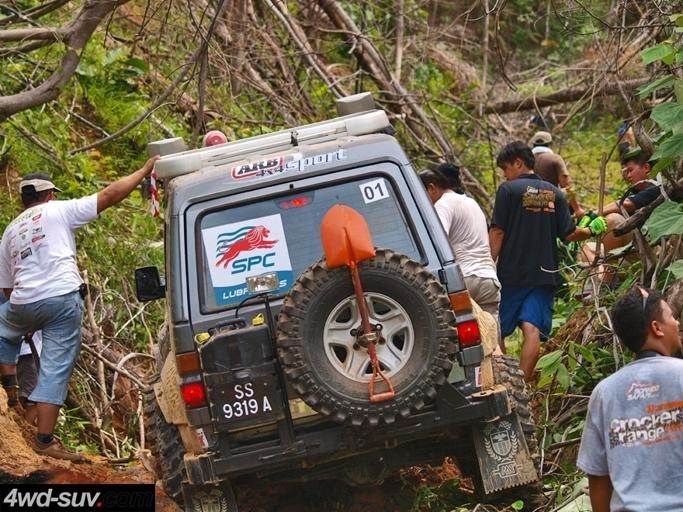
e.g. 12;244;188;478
417;122;683;512
0;154;160;453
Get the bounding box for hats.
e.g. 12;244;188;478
19;173;63;195
533;130;552;145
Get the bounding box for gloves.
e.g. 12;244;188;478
589;216;607;236
577;210;597;227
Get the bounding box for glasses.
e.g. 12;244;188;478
634;283;650;336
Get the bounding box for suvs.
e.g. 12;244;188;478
133;92;541;510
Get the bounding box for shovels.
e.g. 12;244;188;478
319;205;395;403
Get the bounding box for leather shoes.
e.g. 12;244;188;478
5;385;19;407
32;437;82;465
